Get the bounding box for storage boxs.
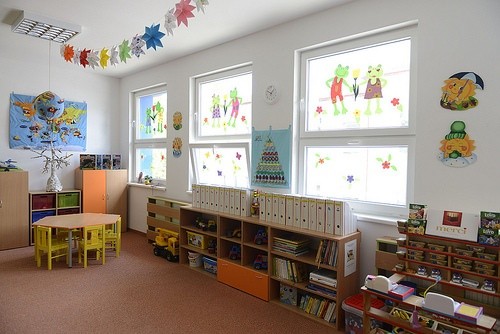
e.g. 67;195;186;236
203;257;218;273
32;209;56;226
369;285;485;334
80;153;122;170
58;193;79;207
32;194;54;209
58;209;79;215
187;231;209;250
279;283;300;306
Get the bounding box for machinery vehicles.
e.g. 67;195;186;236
153;228;180;262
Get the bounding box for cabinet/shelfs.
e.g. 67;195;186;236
391;232;500;298
29;190;81;245
0;167;30;251
360;285;500;334
177;206;362;327
76;170;127;232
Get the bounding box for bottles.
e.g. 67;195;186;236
251;192;259;217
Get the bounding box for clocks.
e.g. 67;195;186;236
264;84;280;106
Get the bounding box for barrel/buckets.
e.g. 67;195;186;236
188;251;202;268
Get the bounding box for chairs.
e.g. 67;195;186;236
34;218;123;270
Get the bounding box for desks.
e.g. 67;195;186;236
33;214;120;268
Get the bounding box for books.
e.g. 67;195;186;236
315;240;338;266
273;257;307;282
300;292;336;323
305;268;337;299
272;235;309;256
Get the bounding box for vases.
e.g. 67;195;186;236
46;164;62;193
